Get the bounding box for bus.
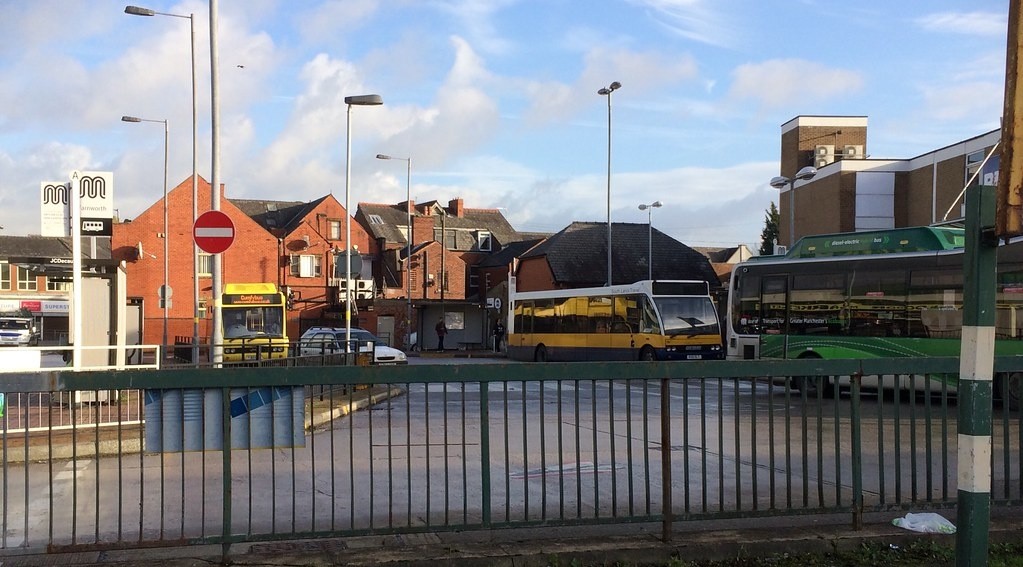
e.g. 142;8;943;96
725;226;1023;410
508;280;724;361
206;282;290;364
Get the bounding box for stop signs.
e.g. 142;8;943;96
192;210;235;254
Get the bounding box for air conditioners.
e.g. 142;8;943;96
813;145;834;169
843;145;863;160
338;279;373;303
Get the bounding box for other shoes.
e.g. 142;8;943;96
436;350;446;353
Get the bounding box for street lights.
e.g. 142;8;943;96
770;167;818;248
638;201;663;280
344;94;384;354
597;81;622;285
121;116;168;362
122;6;200;364
433;206;447;301
375;154;410;351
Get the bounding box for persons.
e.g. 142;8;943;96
492;317;505;353
400;314;417;350
226;313;251;338
435;317;449;353
50;343;73;409
265;314;281;334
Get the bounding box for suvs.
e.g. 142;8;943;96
298;326;408;365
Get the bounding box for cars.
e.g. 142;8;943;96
0;317;40;345
